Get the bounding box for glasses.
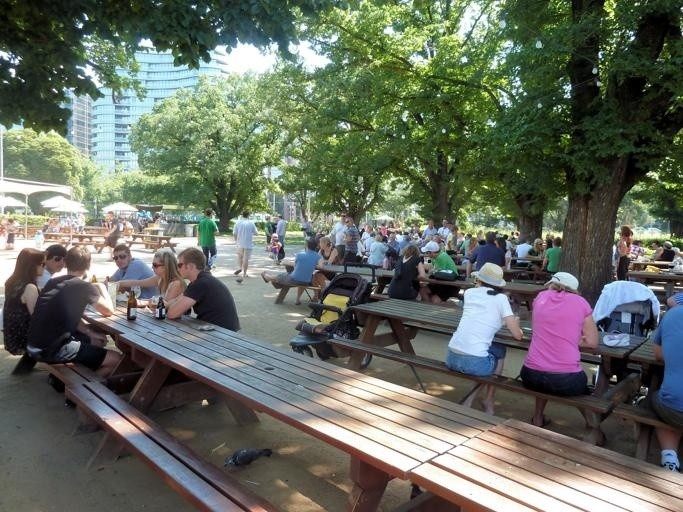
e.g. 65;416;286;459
112;254;130;260
152;263;164;268
177;262;185;268
35;262;46;268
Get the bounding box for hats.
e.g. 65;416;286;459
661;241;672;248
471;262;506;287
543;271;579;291
420;240;440;253
47;245;66;256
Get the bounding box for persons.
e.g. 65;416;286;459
421;241;462;303
301;213;562;277
196;209;219;271
651;305;683;471
388;245;426;301
264;214;287;265
0;209;162;250
611;225;683;310
232;212;258;278
465;231;506;283
3;245;239;407
446;262;523;415
309;237;339;302
261;238;324;305
520;272;607;445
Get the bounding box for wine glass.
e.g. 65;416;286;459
131;285;141;298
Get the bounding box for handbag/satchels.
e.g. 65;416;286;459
603;334;630;347
435;269;457;281
382;284;390;295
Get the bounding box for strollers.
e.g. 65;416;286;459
289;272;372;366
591;279;659;397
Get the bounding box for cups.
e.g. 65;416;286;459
151;295;160;317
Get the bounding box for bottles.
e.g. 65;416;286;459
127;291;137;321
103;275;110;291
155;297;166;320
